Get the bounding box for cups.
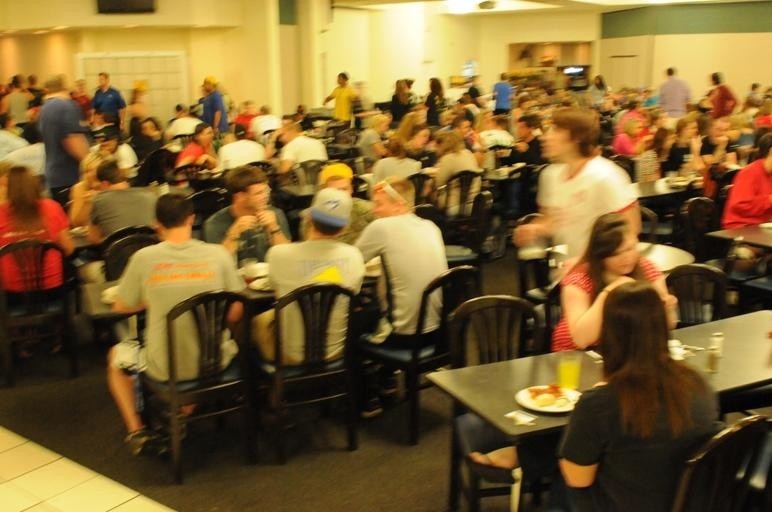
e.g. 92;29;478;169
518;227;548;260
559;348;584;389
243;257;260;282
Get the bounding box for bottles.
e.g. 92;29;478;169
706;332;726;374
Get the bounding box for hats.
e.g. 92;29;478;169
316;161;356;187
313;188;352;226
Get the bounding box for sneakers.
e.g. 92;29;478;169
124;378;396;458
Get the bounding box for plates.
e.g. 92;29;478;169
100;262;272;305
362;254;384;280
513;382;584;416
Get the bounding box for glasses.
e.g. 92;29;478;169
375;181;411;206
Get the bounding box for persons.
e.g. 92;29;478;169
0;46;772;511
